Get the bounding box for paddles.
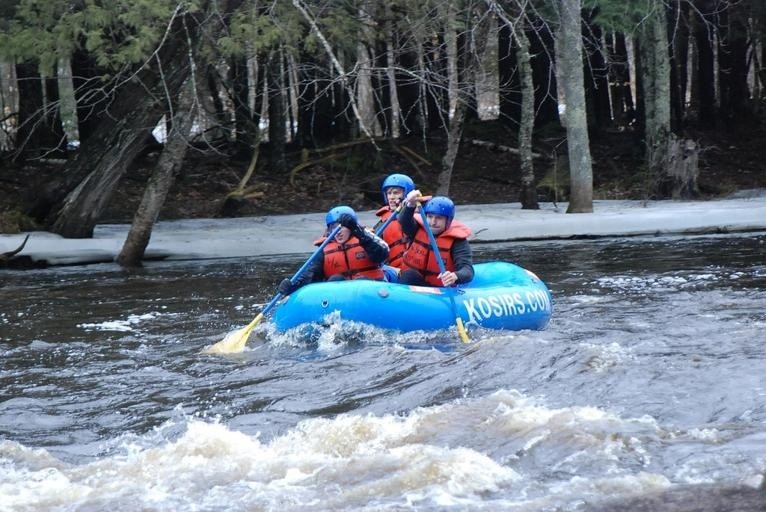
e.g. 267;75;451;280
210;224;342;354
417;202;471;344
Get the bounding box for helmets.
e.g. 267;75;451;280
381;173;415;205
325;205;358;225
423;195;456;232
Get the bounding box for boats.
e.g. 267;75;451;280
273;260;553;332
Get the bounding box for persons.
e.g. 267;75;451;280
399;190;475;288
278;205;392;296
0;106;17;133
370;174;418;283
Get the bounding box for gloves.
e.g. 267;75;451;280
277;277;293;294
337;212;359;232
